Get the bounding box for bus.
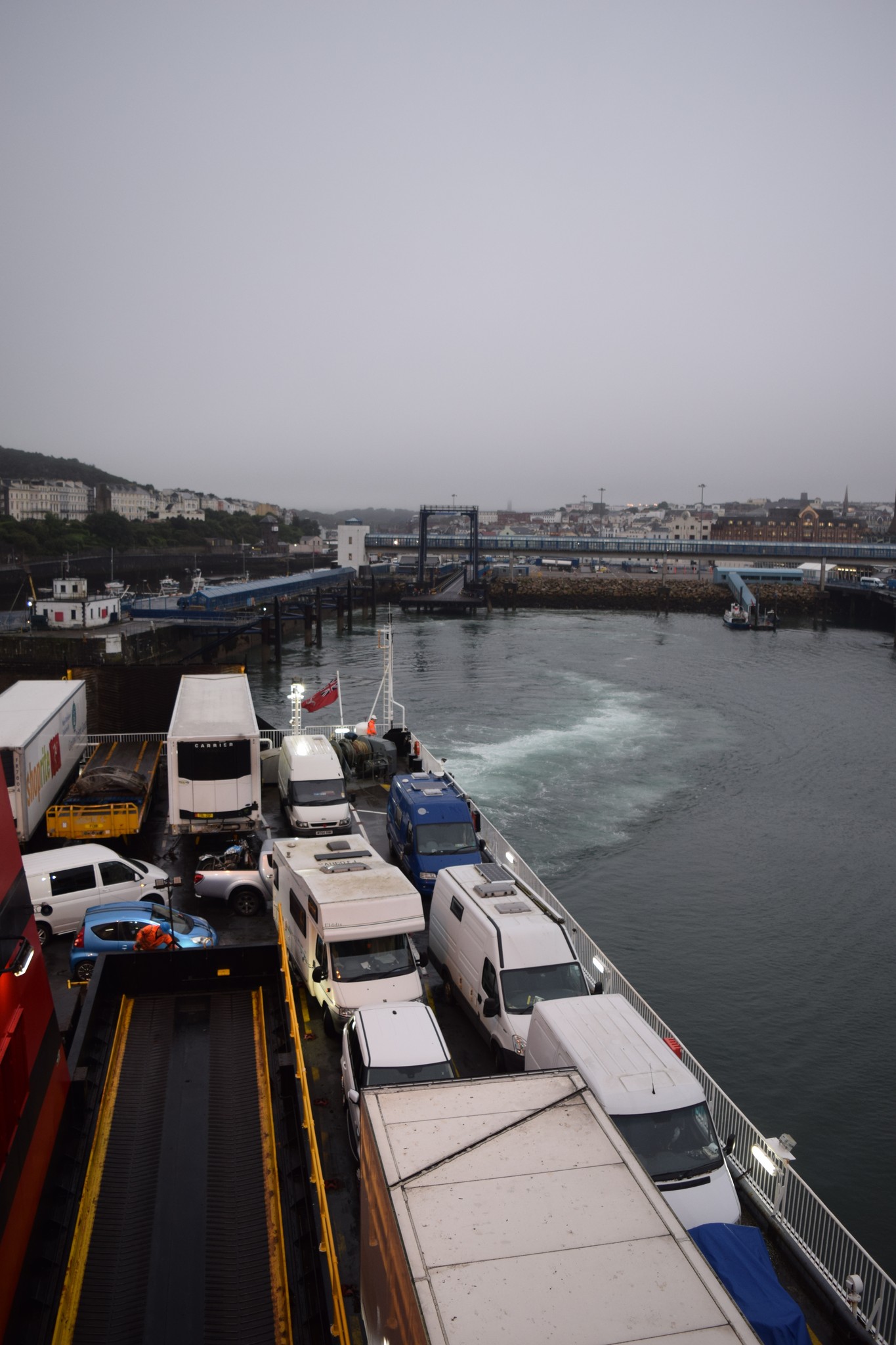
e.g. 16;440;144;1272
0;764;71;1345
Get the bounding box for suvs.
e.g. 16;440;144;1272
339;1000;459;1165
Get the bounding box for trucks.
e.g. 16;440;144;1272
161;673;273;847
272;832;428;1043
356;1066;766;1345
0;679;88;845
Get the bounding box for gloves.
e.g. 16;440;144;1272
135;942;141;950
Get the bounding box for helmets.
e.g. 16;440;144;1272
160;922;170;934
371;715;377;719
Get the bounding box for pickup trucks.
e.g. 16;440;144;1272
193;837;275;918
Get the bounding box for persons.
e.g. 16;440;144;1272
134;922;176;951
367;715;378;737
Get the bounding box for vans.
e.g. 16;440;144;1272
276;734;356;839
387;770;487;895
22;844;173;948
861;576;885;590
428;862;603;1074
524;994;741;1231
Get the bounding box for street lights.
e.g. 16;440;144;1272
598;488;606;549
154;877;184;948
582;495;588;537
452;495;457;512
698;484;707;551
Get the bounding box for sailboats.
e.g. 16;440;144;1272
104;547;124;589
191;553;205;583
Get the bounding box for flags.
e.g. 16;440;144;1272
301;676;339;712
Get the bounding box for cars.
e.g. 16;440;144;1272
68;900;218;982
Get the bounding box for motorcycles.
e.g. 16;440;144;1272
196;833;258;871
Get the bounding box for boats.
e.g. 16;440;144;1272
722;603;751;629
159;576;180;590
